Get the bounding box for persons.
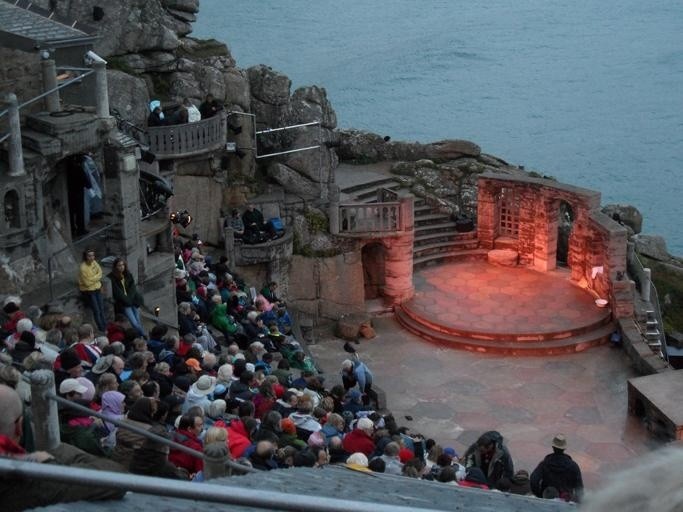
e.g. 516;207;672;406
148;93;222;130
242;204;264;241
458;430;532;496
530;433;583;503
0;210;466;485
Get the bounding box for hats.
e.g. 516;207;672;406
191;375;226;396
186;358;202;371
444;448;454;456
552;433;568;449
3;302;19;312
58;348;113;394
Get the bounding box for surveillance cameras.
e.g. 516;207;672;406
85;50;108;65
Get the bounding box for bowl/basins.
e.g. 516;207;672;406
595;299;608;308
